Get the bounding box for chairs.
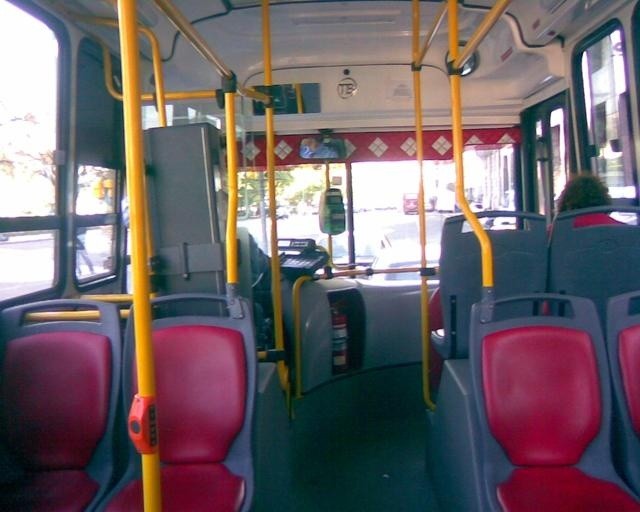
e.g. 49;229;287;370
94;293;257;511
430;210;548;359
607;290;639;494
470;293;639;512
546;201;639;329
2;296;122;511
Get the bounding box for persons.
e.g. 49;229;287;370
299;139;339;158
213;191;285;295
543;174;630;316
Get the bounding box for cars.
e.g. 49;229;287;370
1;232;95;303
277;207;289;220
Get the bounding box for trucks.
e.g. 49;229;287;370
403;192;419;215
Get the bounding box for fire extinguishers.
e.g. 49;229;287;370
329;308;348;366
